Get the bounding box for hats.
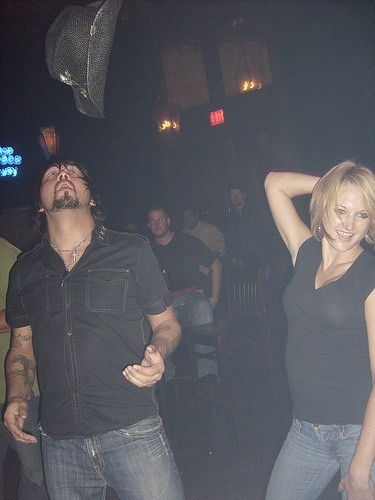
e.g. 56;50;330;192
45;0;126;120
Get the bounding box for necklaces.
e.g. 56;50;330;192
48;233;94;262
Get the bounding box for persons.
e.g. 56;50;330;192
3;157;187;500
0;236;51;500
264;160;375;500
122;186;279;380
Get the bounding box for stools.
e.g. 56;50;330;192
185;273;285;456
